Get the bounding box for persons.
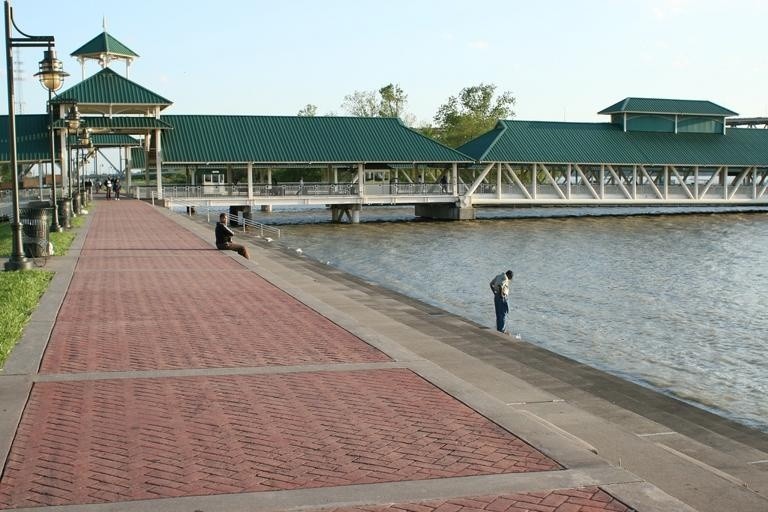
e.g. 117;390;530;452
440;174;449;193
215;212;250;260
489;270;514;335
296;176;304;195
94;174;122;201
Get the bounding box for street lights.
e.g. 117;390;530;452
44;90;97;232
5;0;69;271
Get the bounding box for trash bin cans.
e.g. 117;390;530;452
80;190;86;207
19;206;54;257
57;197;71;228
72;193;81;214
